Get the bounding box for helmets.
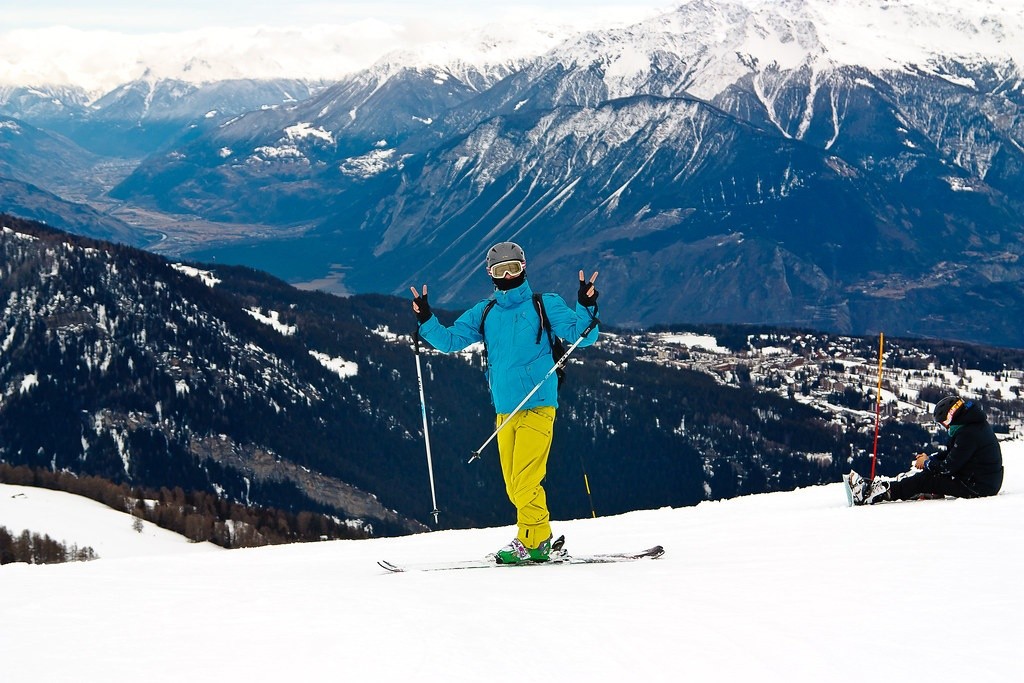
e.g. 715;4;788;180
486;241;525;267
933;396;965;422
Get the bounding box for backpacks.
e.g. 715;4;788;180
478;293;568;389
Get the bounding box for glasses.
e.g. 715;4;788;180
491;261;523;278
940;421;949;429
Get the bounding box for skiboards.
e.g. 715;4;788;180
373;546;665;571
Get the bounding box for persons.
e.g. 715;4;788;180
842;396;1004;507
410;241;599;564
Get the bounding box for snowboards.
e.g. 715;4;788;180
843;474;853;507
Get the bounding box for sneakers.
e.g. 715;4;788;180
850;472;870;505
496;539;550;564
